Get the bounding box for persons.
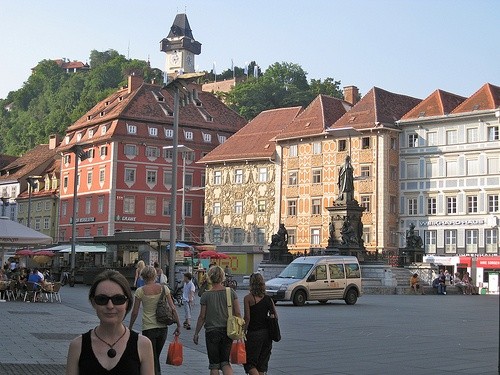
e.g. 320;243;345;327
276;224;288;246
338;221;356;245
337;156;355;201
410;223;415;235
0;259;281;375
411;269;479;295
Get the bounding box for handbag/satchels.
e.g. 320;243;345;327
265;294;281;342
155;284;175;326
165;334;183;367
226;313;248;340
229;339;247;365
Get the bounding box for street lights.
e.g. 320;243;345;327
69;144;90;287
25;176;38;271
161;75;191;296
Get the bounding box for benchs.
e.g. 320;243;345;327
422;285;449;295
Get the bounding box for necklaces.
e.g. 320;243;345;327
94;324;126;357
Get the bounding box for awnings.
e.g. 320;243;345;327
35;245;107;252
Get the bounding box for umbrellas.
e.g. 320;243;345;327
168;243;228;267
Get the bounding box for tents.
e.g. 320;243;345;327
0;219;53;269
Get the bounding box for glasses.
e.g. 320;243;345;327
92;293;128;306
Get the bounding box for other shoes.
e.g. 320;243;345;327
183;321;192;331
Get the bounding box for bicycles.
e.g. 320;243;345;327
167;280;184;308
221;274;237;290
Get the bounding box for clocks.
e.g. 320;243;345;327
185;55;192;66
171;54;179;66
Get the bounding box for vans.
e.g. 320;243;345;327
264;256;362;306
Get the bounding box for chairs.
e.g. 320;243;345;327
0;278;63;303
